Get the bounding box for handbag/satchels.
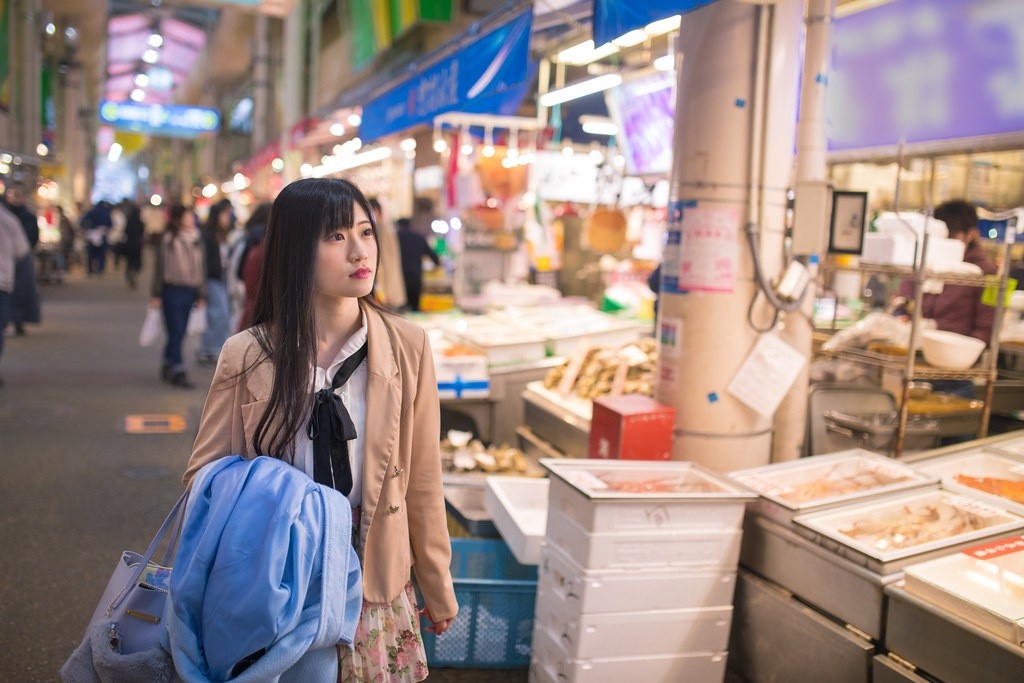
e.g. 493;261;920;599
59;488;184;683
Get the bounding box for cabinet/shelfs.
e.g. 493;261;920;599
821;232;1014;456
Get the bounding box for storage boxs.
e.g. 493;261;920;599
410;207;1024;683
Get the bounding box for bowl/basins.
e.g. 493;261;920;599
921;329;986;369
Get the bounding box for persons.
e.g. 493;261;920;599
370;197;441;312
182;177;459;683
0;182;40;337
893;199;998;448
147;199;275;390
54;198;146;287
